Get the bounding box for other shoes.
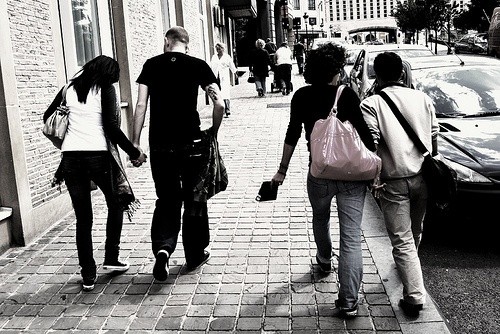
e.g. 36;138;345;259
398;299;423;311
186;249;211;272
282;86;286;95
335;299;358;316
315;251;332;272
152;248;170;284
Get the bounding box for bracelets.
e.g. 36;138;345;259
279;163;288;176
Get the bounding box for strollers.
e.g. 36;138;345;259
270;64;293;93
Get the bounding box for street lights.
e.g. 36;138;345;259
329;25;333;38
303;12;310;51
310;19;314;40
320;23;325;38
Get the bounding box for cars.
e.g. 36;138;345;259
343;45;365;83
348;44;436;102
454;33;489;57
361;54;500;246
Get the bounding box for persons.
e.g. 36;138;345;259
43;56;147;290
130;26;225;280
360;51;440;312
211;42;238;117
250;38;306;97
272;43;377;316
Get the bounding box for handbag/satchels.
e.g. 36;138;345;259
422;154;457;209
310;85;382;181
247;71;256;83
41;84;71;150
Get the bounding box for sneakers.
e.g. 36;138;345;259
82;273;98;290
102;260;130;272
